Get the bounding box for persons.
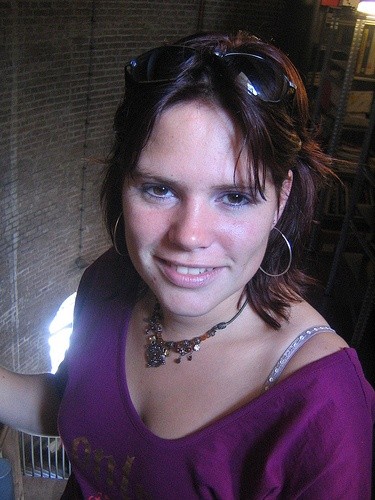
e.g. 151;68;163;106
0;34;375;500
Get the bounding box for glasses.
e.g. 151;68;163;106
122;44;297;106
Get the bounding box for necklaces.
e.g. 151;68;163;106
144;297;250;368
